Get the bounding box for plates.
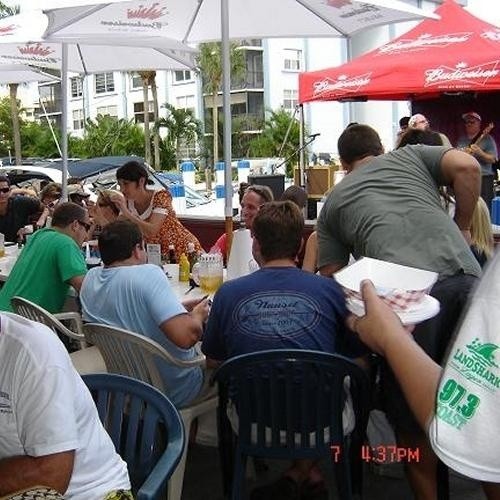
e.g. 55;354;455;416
345;293;441;327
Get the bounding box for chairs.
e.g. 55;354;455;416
0;217;491;500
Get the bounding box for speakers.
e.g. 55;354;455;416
247;174;284;201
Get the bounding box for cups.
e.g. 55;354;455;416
0;233;5;259
146;244;163;267
202;255;224;289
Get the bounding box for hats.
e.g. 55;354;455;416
462;112;481;120
92;183;124;212
67;183;91;197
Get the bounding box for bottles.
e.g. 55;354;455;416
168;240;199;282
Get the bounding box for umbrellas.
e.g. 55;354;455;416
0;66;61;87
43;1;440;271
0;12;198;201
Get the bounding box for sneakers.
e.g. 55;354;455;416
250;475;329;500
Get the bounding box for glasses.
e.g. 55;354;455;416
0;188;10;192
77;219;92;232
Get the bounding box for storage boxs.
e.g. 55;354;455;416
292;163;340;201
246;172;287;202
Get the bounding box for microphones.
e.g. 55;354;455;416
307;133;320;137
